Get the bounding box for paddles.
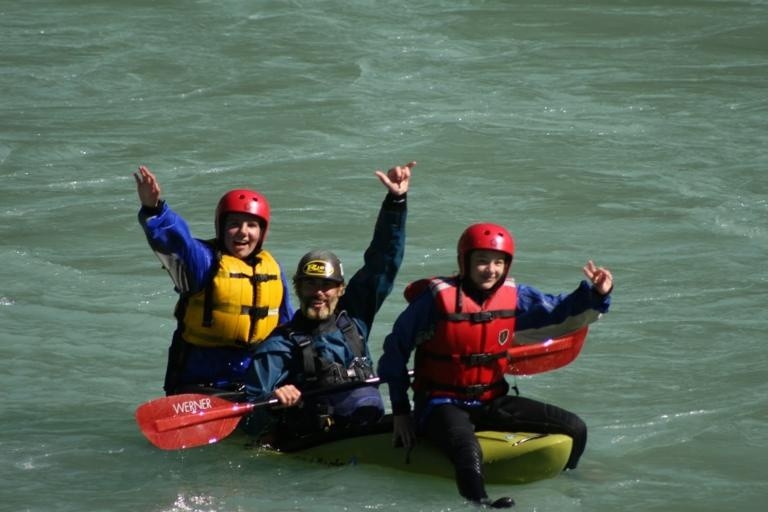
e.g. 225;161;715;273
136;326;587;451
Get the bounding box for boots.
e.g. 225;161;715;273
453;471;518;509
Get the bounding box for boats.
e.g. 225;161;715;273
226;413;575;485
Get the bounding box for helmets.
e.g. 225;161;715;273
215;187;270;245
293;251;346;283
457;224;515;261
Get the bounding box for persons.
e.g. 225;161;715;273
377;224;614;509
243;160;418;453
130;164;296;398
